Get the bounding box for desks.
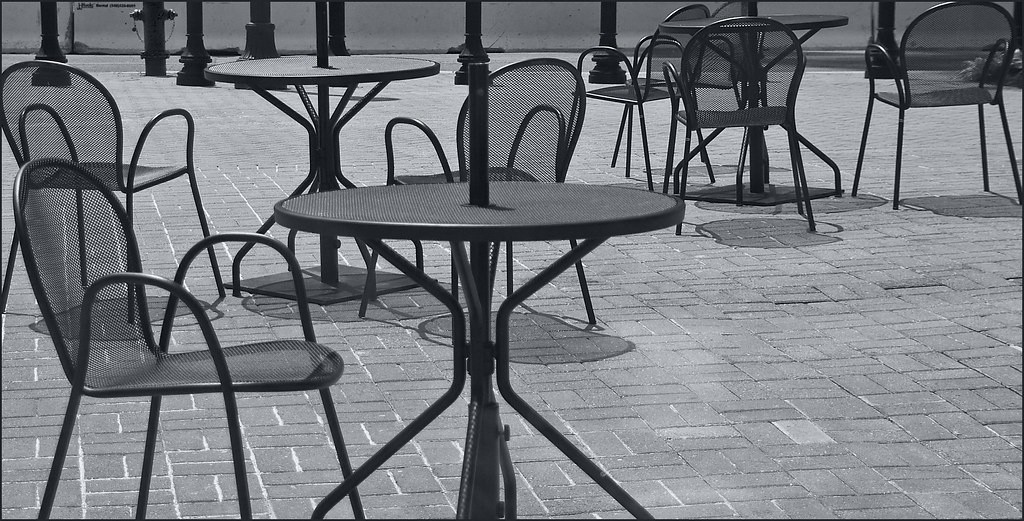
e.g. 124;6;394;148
274;181;686;520
204;55;440;306
657;16;849;206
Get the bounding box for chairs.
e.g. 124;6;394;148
851;1;1022;211
11;157;364;520
662;16;818;234
572;3;717;184
1;59;226;323
358;58;597;336
